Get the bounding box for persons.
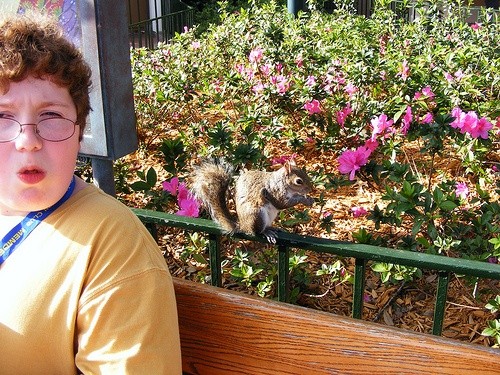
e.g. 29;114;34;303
0;2;182;375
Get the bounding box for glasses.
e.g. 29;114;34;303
0;117;83;144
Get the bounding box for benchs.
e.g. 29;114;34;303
172;276;500;375
